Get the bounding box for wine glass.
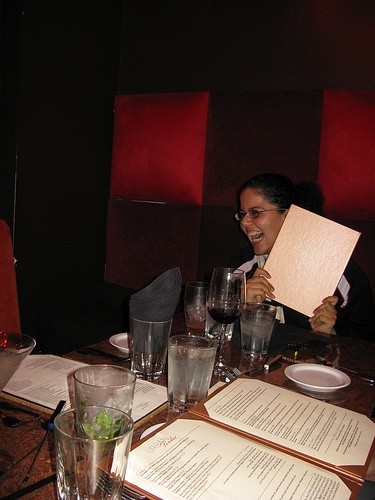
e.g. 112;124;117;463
207;267;247;376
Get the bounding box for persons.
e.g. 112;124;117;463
232;172;375;335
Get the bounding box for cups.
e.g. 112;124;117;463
166;335;218;421
240;302;277;362
54;406;134;500
130;315;172;382
73;364;137;424
184;281;209;345
0;331;36;391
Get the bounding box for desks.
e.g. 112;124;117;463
0;316;375;500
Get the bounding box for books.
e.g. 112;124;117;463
0;354;170;438
96;374;375;500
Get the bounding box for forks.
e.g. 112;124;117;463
96;468;150;500
313;353;374;384
81;345;130;362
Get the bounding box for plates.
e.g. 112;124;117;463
109;332;130;354
284;363;351;392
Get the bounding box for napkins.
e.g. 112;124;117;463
129;267;183;359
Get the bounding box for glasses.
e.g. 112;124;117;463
235;208;288;220
